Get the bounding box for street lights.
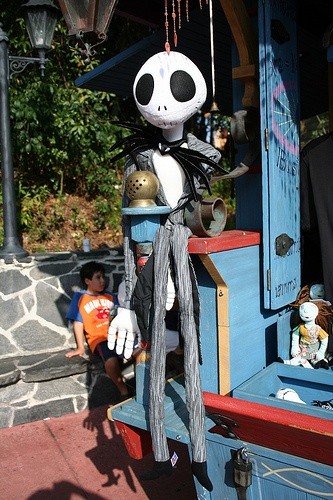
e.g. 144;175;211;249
0;0;62;258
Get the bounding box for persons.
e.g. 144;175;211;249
276;302;330;371
64;261;139;399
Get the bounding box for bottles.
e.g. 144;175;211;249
133;340;151;405
83;234;90;252
